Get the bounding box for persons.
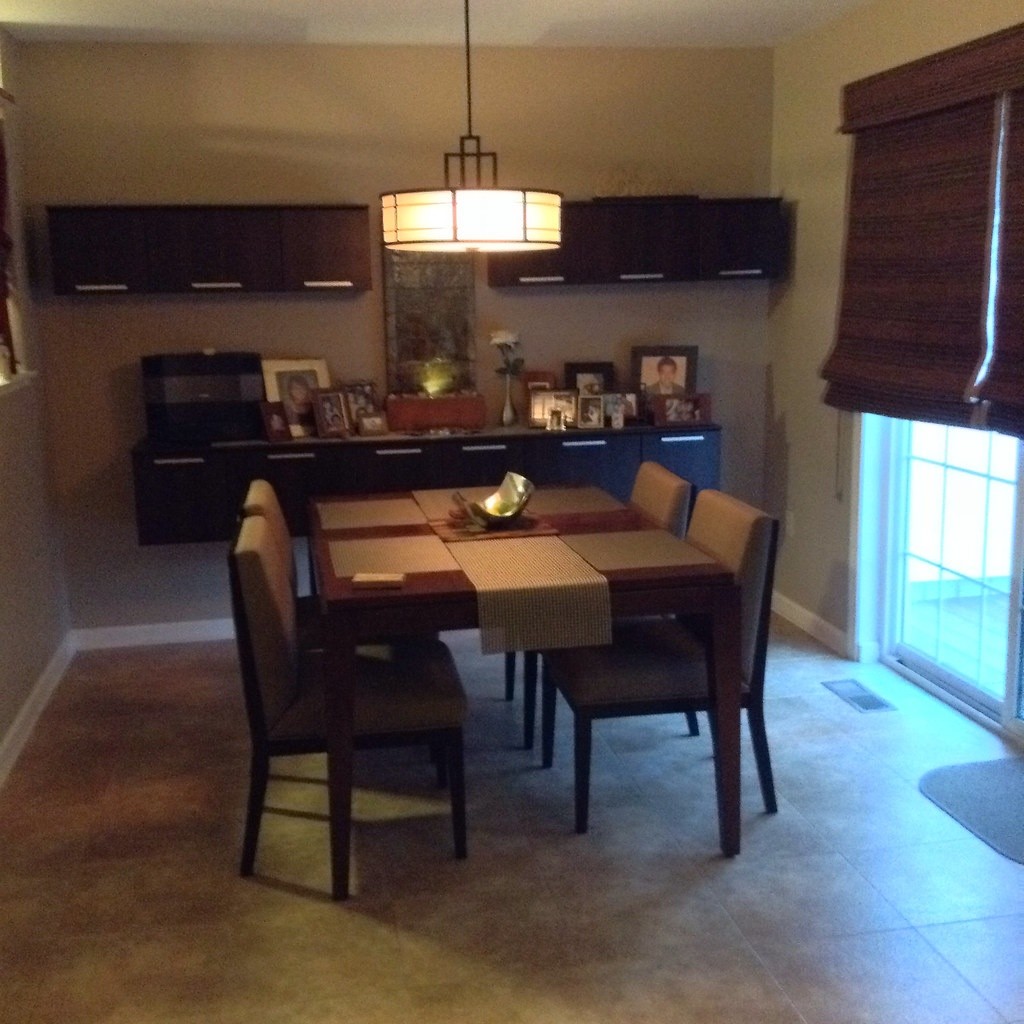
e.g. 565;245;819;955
644;357;684;394
583;395;634;424
286;374;369;431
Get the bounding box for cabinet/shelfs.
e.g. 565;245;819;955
42;202;376;293
264;432;723;535
486;193;791;285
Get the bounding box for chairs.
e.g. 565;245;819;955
542;486;780;832
504;456;697;749
225;516;470;880
243;479;437;761
129;349;270;546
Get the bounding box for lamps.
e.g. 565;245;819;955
379;0;563;253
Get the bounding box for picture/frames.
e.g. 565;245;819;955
275;369;388;439
521;345;712;432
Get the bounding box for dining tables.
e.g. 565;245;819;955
300;482;743;899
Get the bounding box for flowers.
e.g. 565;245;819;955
488;329;523;376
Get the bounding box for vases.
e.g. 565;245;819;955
502;374;515;425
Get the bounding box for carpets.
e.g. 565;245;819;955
919;754;1024;865
